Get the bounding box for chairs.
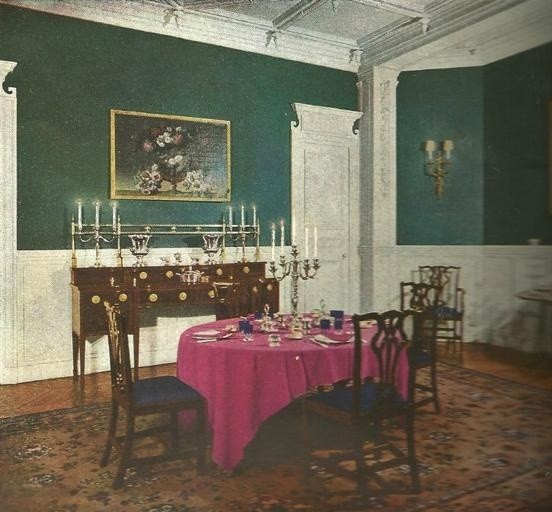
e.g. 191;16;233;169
100;299;209;490
301;306;422;495
397;263;467;413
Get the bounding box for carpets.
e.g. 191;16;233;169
1;358;551;512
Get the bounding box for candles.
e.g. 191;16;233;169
70;199;123;234
227;205;256;226
269;218;320;262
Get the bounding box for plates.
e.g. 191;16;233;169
350;325;375;329
192;329;220;337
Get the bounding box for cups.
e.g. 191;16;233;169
319;318;343;337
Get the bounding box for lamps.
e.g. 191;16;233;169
425;138;455;200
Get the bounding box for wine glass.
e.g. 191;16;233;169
238;320;254;343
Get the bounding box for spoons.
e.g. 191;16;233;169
191;333;233;340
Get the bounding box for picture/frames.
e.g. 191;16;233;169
107;109;232;202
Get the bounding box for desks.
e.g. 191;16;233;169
69;263;279;400
